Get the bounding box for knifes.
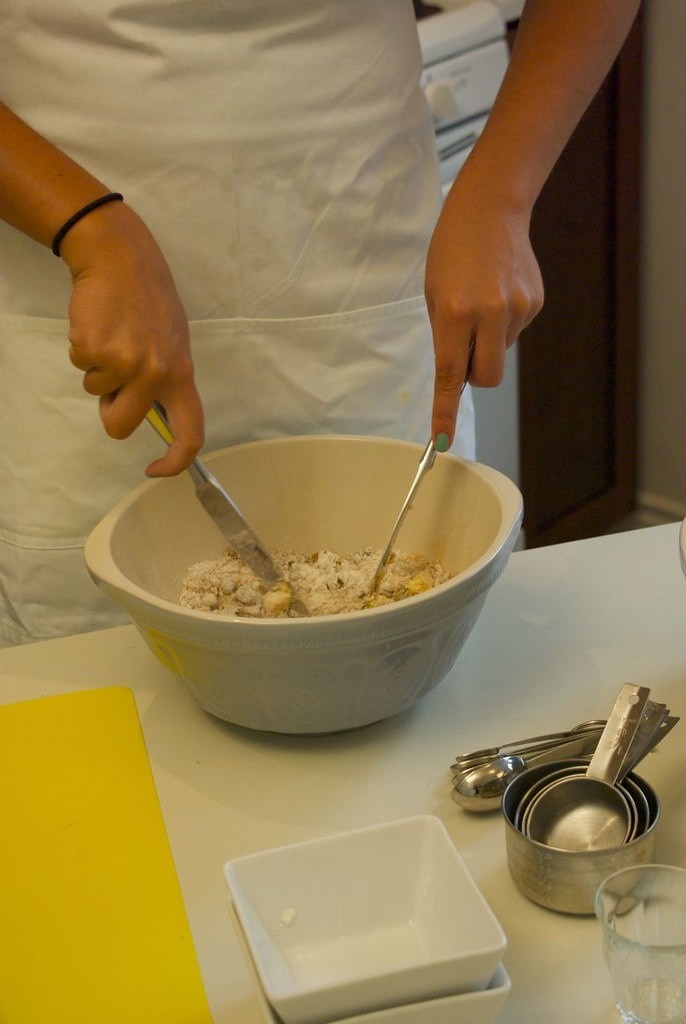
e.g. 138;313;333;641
364;341;476;604
147;404;310;616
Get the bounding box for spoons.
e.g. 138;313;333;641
451;719;607;813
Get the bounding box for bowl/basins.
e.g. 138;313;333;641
221;815;511;1024
502;761;659;914
83;433;523;733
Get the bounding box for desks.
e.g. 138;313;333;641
0;519;686;1024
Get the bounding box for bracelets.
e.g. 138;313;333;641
52;192;122;255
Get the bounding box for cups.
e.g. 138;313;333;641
594;863;686;1024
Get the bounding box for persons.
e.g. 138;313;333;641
0;1;648;650
